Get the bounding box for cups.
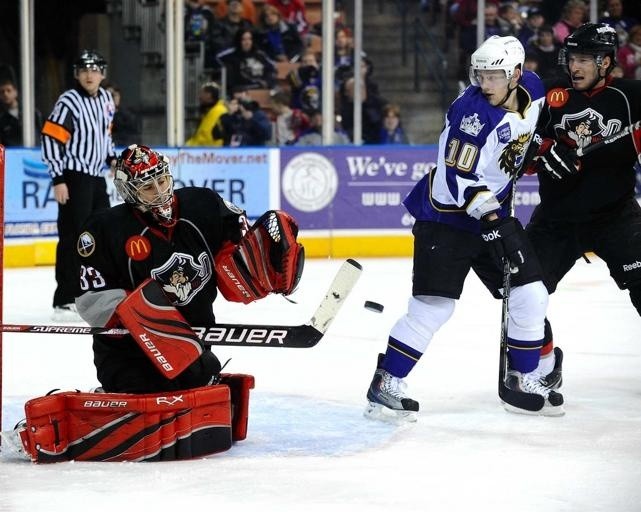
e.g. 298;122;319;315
474;73;505;83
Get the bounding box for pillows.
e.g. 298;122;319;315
557;21;620;93
114;143;175;220
467;32;526;108
73;49;108;80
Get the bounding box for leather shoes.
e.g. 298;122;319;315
539;137;583;184
481;215;532;273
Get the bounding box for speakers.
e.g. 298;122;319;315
366;353;419;413
501;346;565;407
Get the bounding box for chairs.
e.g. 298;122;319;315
124;0;641;147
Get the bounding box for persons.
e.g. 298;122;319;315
15;146;304;462
368;36;563;412
0;80;42;148
432;0;640;82
524;23;640;392
42;52;120;310
105;82;142;148
176;1;408;143
